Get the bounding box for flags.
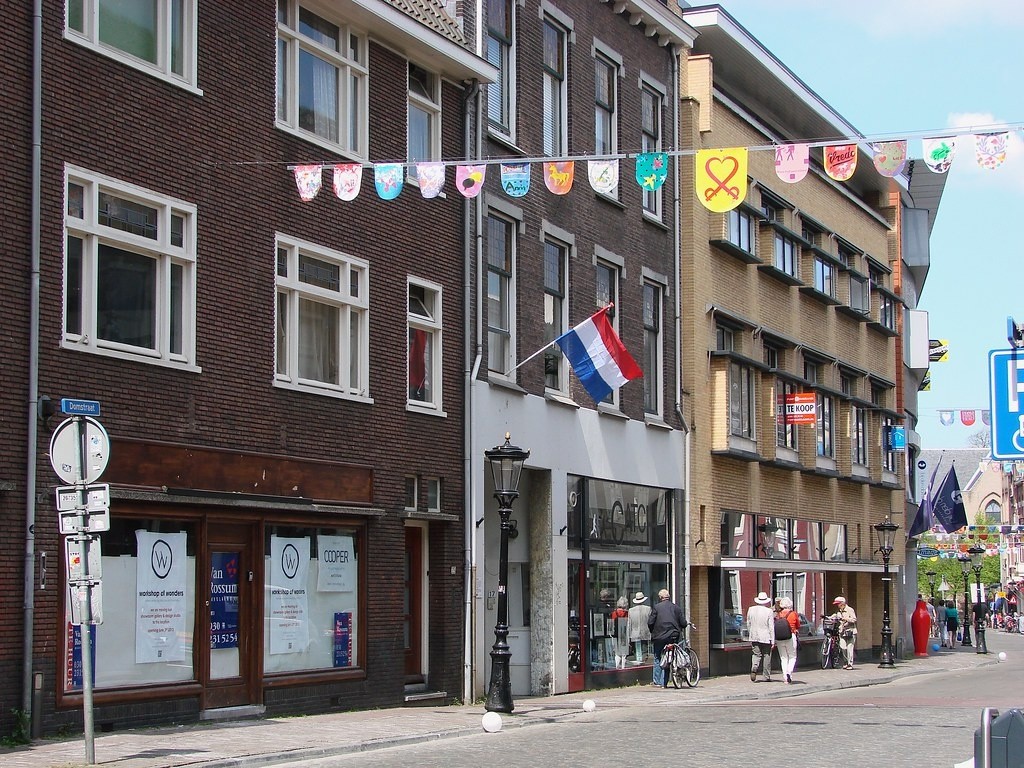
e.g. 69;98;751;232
908;465;939;538
555;308;643;403
932;467;968;534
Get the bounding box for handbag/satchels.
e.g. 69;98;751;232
659;643;690;670
957;629;961;641
840;630;853;638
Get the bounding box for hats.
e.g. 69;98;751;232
632;592;648;604
833;596;846;605
754;592;772;604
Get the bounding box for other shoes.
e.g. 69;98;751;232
751;666;758;681
654;682;661;686
846;664;852;670
843;662;848;668
785;674;791;683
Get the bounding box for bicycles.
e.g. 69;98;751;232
666;622;700;689
820;614;842;669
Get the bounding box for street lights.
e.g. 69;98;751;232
991;585;997;629
925;571;937;605
958;554;972;646
484;432;531;715
966;544;993;654
874;515;901;669
1008;579;1016;632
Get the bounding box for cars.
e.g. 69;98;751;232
957;610;965;625
265;584;318;640
798;613;818;638
724;609;749;637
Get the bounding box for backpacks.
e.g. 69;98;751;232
612;611;628;637
774;610;794;640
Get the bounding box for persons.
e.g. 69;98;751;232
647;589;688;688
627;592;652;662
944;601;960;649
747;591;775;682
936;600;949;647
771;597;783;618
927;600;937;619
612;596;629;669
774;597;800;684
826;596;857;670
